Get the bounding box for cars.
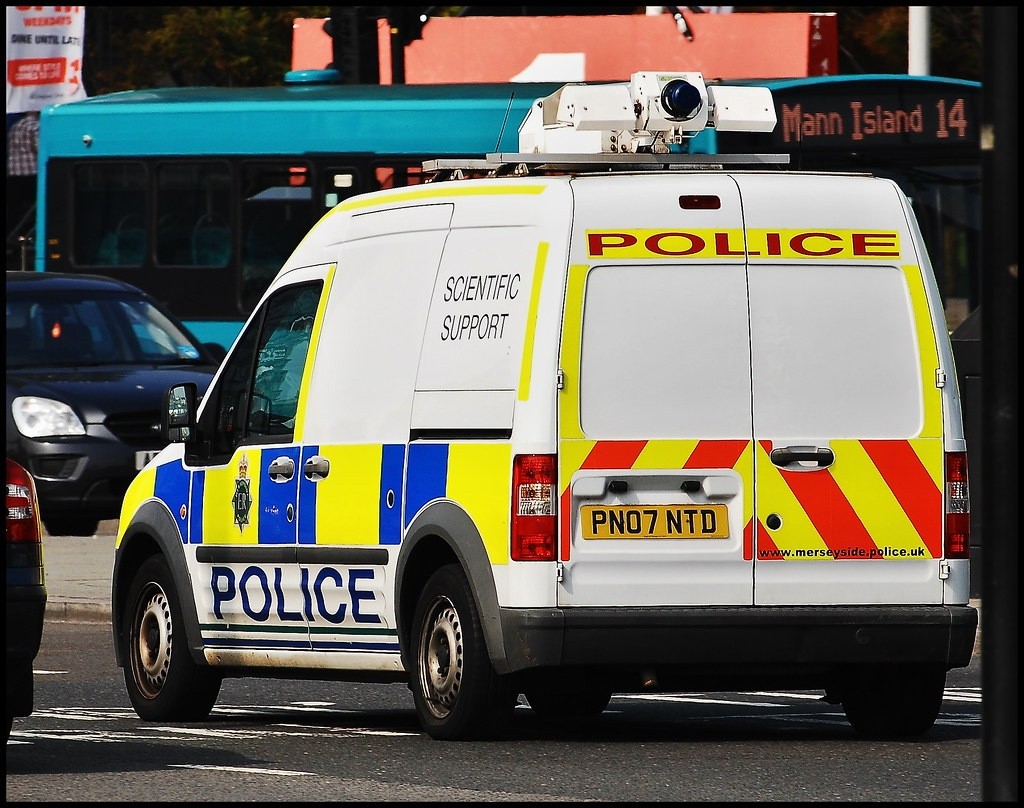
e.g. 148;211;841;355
1;269;237;538
5;456;47;766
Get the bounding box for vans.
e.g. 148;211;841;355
108;66;979;741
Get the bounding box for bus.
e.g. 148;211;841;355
26;57;989;463
